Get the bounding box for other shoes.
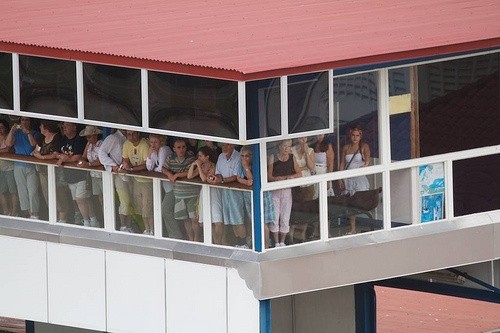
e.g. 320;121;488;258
120;226;135;233
2;207;81;225
234;244;249;248
83;220;90;227
143;230;154;235
289;228;358;248
91;219;100;227
275;242;287;247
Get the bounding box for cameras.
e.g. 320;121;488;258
119;164;124;169
210;176;215;181
16;124;23;129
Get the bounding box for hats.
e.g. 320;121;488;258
79;125;103;137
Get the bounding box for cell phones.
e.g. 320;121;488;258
78;161;83;164
35;144;41;151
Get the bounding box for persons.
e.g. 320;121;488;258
303;132;337;239
0;107;277;258
340;128;379;236
269;139;302;249
290;135;316;243
